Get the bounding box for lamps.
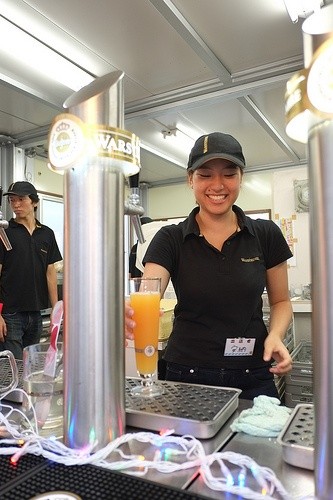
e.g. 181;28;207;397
162;128;195;155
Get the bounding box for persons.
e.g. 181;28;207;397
0;181;64;360
124;132;294;401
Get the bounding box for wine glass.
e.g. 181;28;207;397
130;277;165;397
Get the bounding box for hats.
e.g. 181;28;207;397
187;132;246;172
3;181;38;197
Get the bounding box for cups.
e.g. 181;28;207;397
23;342;66;439
